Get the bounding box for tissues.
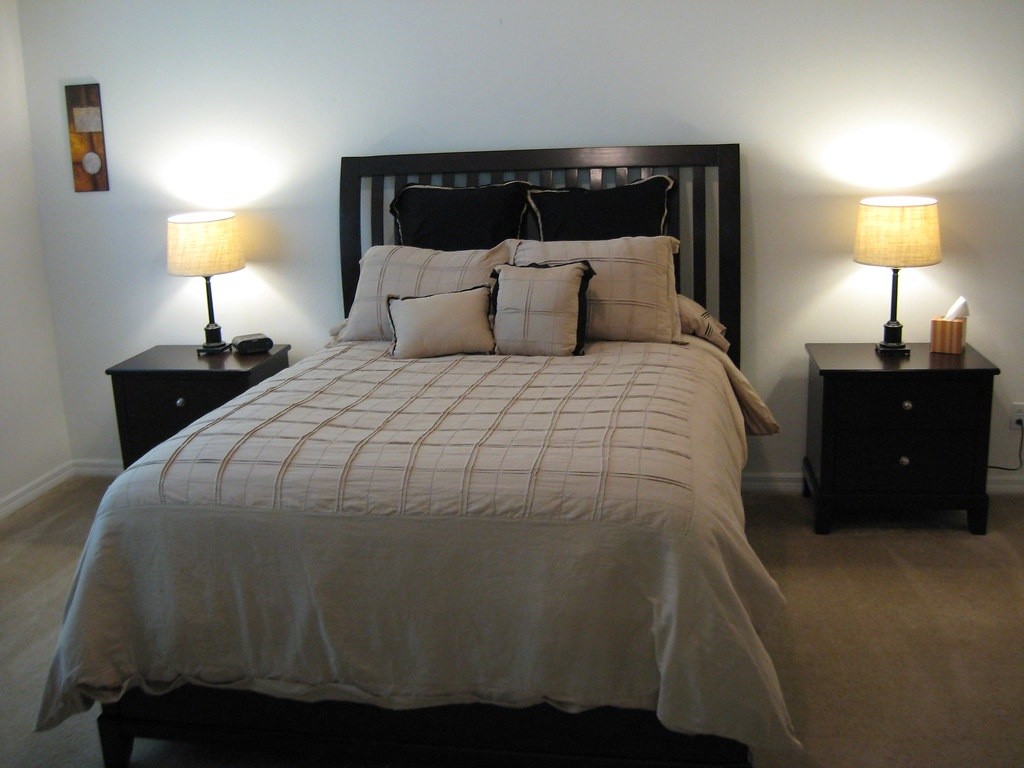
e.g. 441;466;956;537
930;296;970;355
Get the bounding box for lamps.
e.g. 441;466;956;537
167;210;246;356
853;196;942;356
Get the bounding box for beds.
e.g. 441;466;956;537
31;143;805;768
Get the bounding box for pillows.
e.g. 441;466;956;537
526;175;673;242
389;180;534;252
490;259;596;356
512;236;690;344
333;238;521;342
385;284;496;359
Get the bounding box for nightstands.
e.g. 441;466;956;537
104;344;292;468
800;343;1000;535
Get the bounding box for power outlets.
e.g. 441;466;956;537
1009;402;1024;430
